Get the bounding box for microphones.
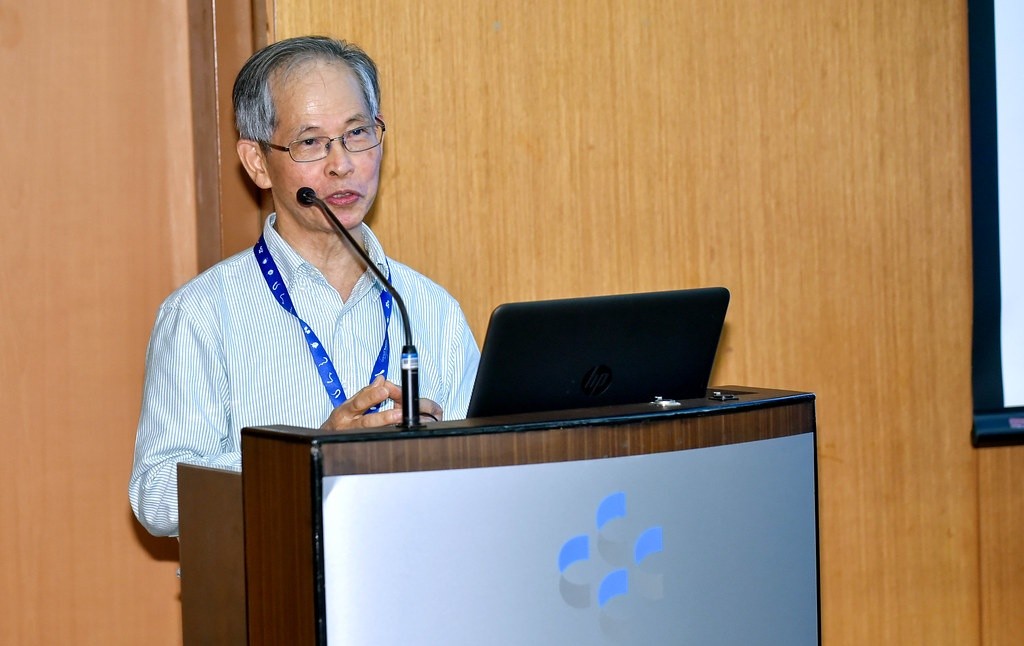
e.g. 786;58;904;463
297;187;425;428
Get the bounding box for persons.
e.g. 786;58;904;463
129;37;481;538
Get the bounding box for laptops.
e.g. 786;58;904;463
466;287;730;420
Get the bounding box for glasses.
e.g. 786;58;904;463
259;117;386;163
